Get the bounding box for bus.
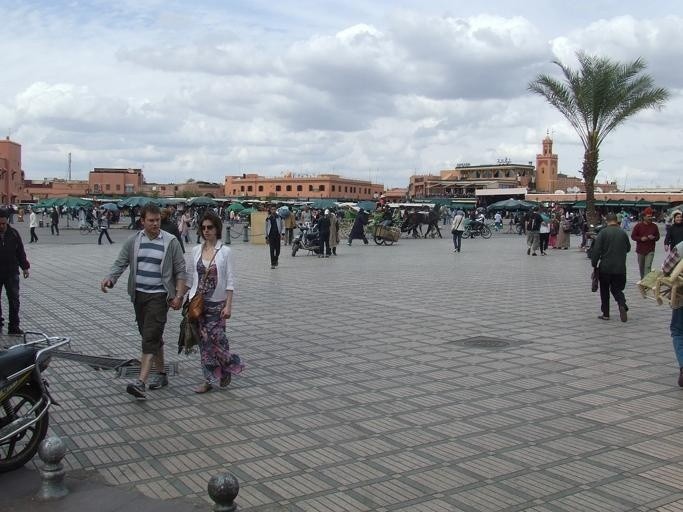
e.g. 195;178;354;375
384;200;434;219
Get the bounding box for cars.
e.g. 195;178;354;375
0;202;71;214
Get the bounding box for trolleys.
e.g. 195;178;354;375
368;223;399;246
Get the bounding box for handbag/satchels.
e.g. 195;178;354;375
590;268;598;292
182;293;206;317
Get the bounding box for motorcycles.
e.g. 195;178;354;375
0;329;74;474
584;222;602;259
461;223;492;239
289;222;320;256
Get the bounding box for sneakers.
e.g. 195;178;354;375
148;374;169;389
598;315;610;320
7;328;25;336
126;380;146;400
528;248;547;256
620;305;627;322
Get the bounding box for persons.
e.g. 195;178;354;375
591;214;631;322
172;213;245;394
662;240;683;387
7;195;676;259
101;204;186;400
0;208;30;334
664;210;683;253
265;206;285;269
631;207;660;289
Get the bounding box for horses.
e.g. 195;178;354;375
409;204;452;238
401;203;442;239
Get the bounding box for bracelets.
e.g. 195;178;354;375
177;295;184;300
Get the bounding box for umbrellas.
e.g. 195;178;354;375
667;205;683;213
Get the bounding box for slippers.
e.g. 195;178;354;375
195;385;210;392
220;372;231;387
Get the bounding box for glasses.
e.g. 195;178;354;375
200;225;214;230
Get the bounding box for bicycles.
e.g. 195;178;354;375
78;222;101;236
